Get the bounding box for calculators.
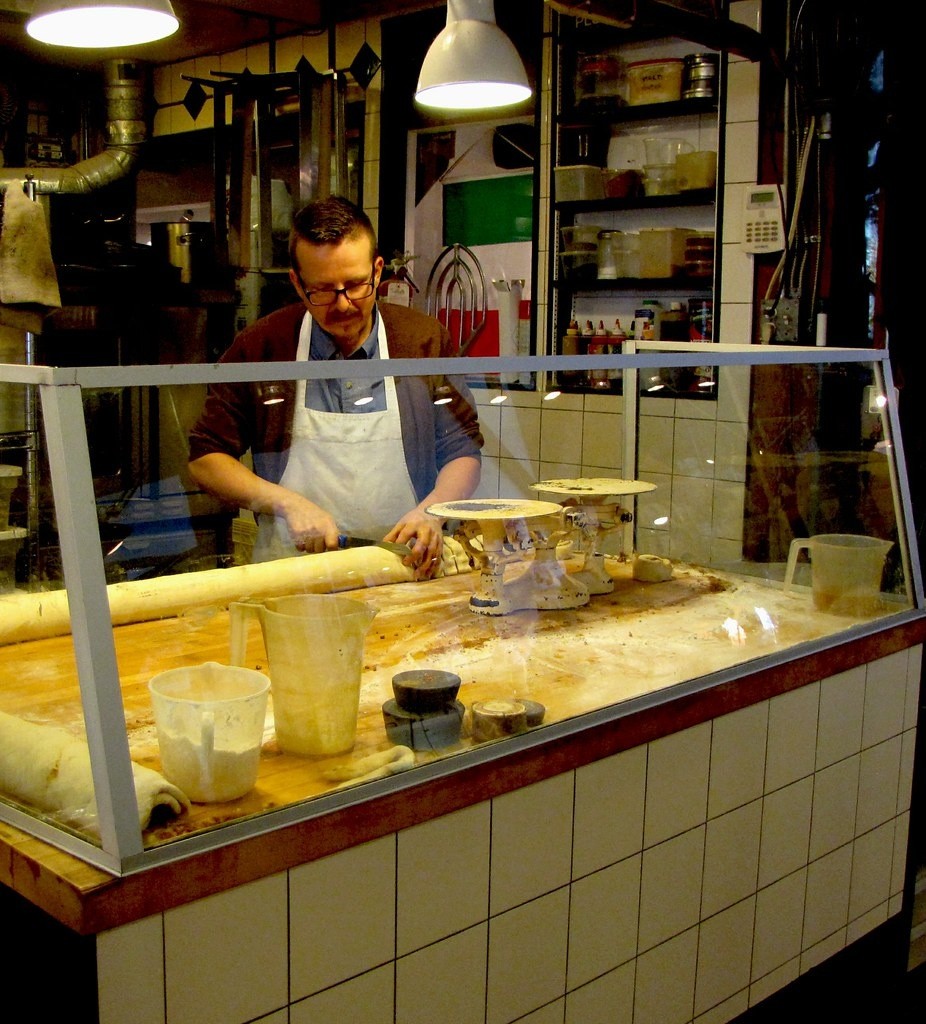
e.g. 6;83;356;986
741;185;786;255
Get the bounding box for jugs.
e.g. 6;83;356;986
147;661;270;802
224;587;379;757
784;533;896;615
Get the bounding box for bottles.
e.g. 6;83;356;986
596;230;621;280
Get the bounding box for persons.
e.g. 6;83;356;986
187;193;485;581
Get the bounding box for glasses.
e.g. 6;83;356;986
294;262;375;306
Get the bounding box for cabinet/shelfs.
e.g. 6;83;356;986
545;0;729;399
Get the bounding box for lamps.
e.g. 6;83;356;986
412;0;534;115
24;0;180;49
